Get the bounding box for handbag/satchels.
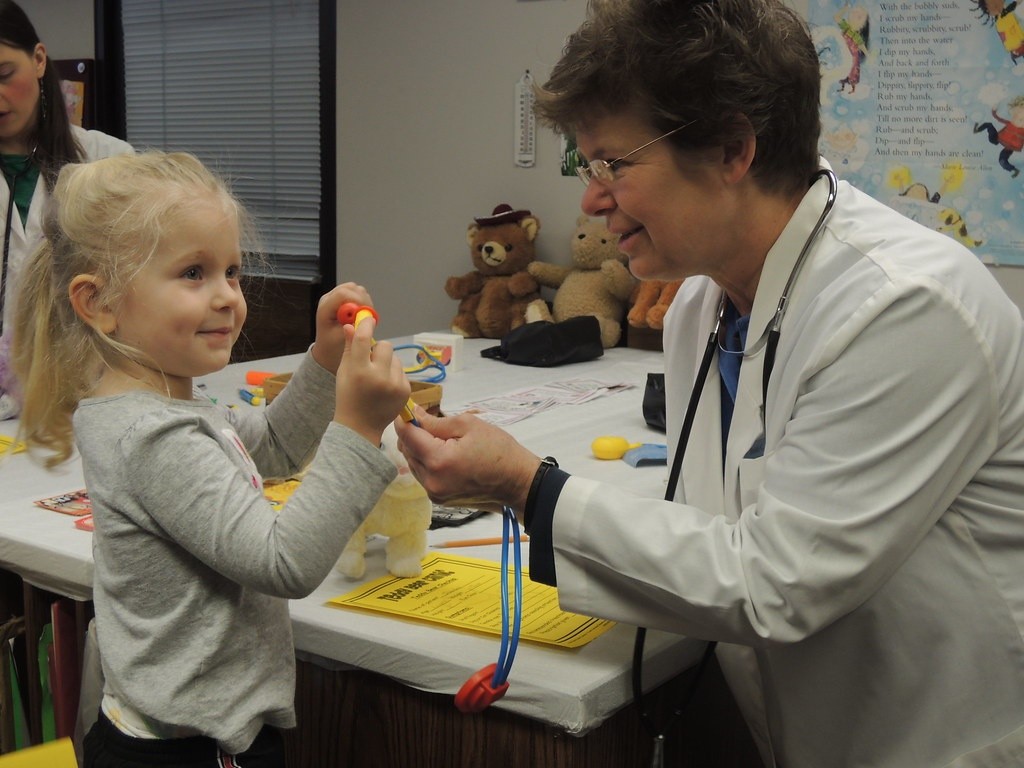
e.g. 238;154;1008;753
481;316;605;369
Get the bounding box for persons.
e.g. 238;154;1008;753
0;0;413;768
374;0;1024;768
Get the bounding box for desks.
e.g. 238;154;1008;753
0;330;712;768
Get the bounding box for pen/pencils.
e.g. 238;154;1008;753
425;535;532;549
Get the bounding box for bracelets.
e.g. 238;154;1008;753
518;457;558;529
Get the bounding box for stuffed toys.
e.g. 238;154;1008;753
444;202;682;346
336;419;432;579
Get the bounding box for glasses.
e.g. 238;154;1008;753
575;112;714;187
